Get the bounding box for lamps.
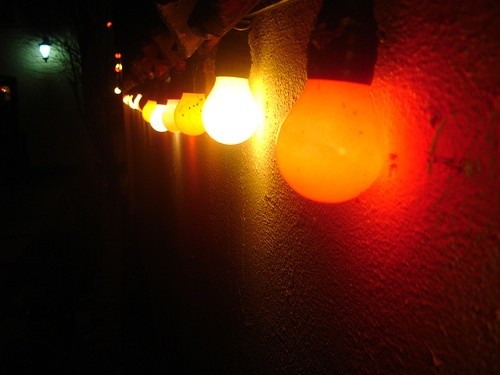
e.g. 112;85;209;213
38;36;52;63
